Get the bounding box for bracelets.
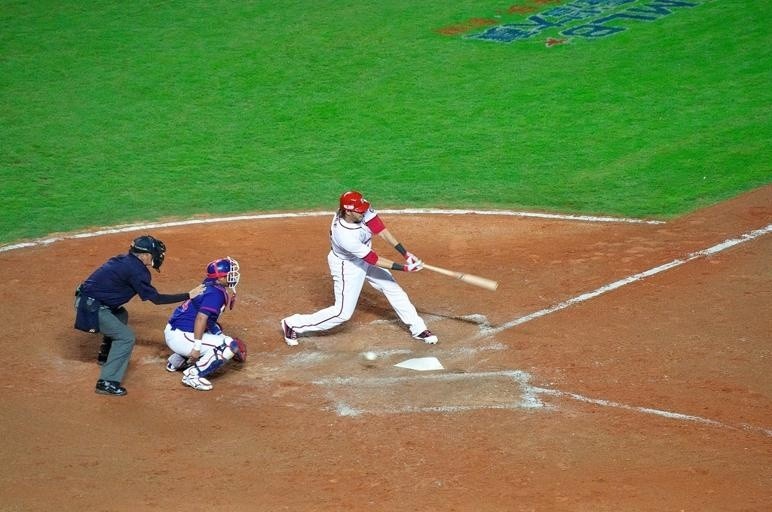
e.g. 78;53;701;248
192;339;202;350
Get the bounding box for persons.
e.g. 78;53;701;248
163;255;241;392
280;191;438;347
74;235;208;396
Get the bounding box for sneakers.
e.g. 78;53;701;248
280;319;298;346
182;369;213;391
95;381;127;396
415;330;438;344
166;359;192;372
98;352;108;364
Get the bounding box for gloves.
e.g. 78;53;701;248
403;252;419;264
403;259;425;272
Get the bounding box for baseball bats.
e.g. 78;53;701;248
422;264;498;291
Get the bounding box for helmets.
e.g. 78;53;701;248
340;191;370;215
129;235;166;272
204;256;240;287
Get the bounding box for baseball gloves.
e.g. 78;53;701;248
231;338;246;363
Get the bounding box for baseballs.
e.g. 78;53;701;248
367;352;376;361
